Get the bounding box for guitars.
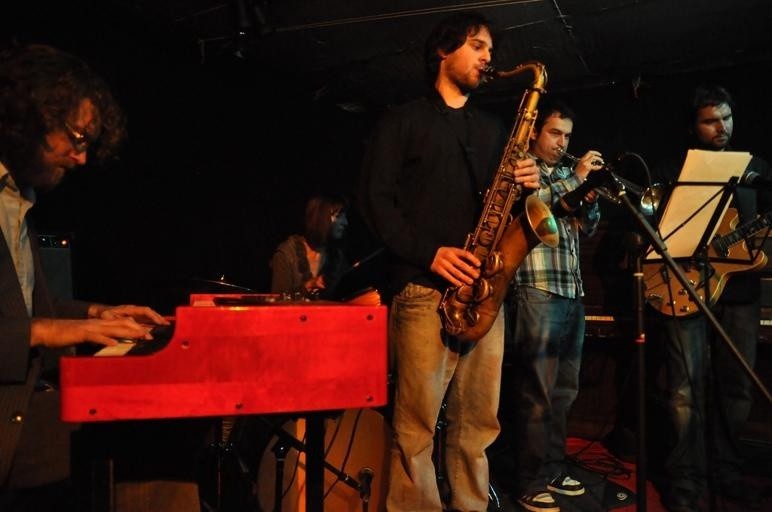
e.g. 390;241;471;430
640;209;772;317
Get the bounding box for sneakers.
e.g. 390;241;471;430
514;488;561;512
547;471;586;498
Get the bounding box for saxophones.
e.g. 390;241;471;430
439;63;560;338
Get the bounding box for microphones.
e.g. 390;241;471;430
551;153;624;218
356;467;373;504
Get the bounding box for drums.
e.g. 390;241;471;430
256;410;392;512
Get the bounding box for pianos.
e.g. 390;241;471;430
62;294;387;421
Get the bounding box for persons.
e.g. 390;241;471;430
362;11;543;511
263;184;368;301
1;40;171;510
507;96;608;510
648;81;772;511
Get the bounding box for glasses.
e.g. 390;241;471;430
58;116;91;153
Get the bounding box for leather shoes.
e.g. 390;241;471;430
716;477;770;510
658;482;701;512
